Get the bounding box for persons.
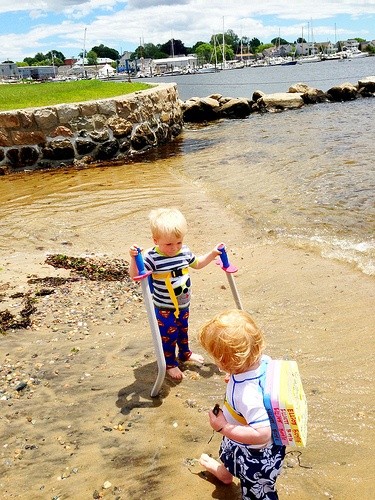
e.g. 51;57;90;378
198;309;286;500
129;207;227;380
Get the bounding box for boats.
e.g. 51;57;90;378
138;68;220;78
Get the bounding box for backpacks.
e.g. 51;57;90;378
223;356;308;448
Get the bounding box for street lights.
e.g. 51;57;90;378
82;27;87;73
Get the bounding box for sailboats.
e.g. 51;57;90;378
214;13;370;69
